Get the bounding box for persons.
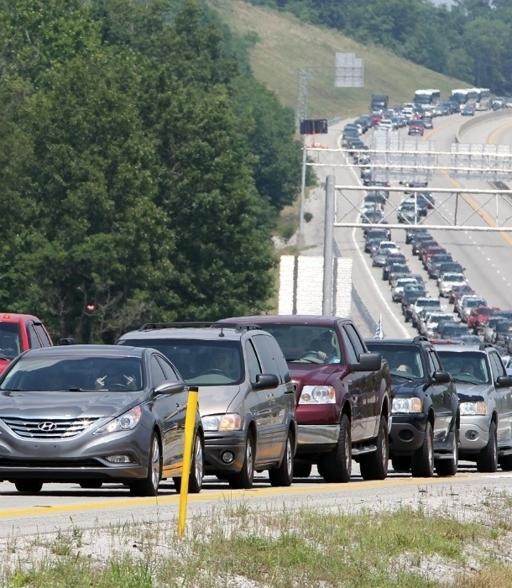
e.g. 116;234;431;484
460;361;476;378
93;357;139;394
205;347;234;377
302;329;335;362
392;350;415;373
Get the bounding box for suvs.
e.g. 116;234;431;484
366;336;458;474
212;316;392;476
118;320;299;490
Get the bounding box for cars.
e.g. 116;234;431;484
341;151;436;224
434;346;512;471
0;313;52;384
0;344;206;497
451;86;512;116
358;227;511;346
341;82;461;150
